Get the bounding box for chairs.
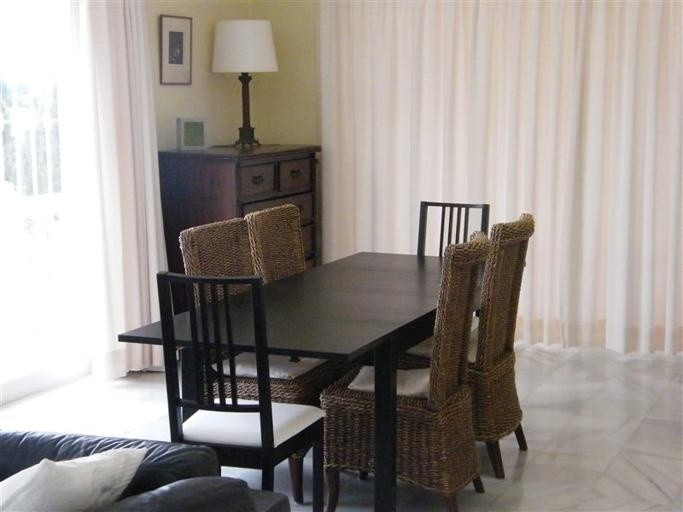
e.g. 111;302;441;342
176;217;355;502
399;213;534;478
398;201;491;368
320;230;489;512
241;202;360;386
156;272;328;512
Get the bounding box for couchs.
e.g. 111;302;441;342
0;429;292;511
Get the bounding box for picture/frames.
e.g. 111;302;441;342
158;16;193;85
179;117;208;150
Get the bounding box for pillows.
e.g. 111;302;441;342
1;448;147;511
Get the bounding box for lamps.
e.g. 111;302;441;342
209;18;277;150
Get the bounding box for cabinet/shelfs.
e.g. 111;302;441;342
160;143;322;312
